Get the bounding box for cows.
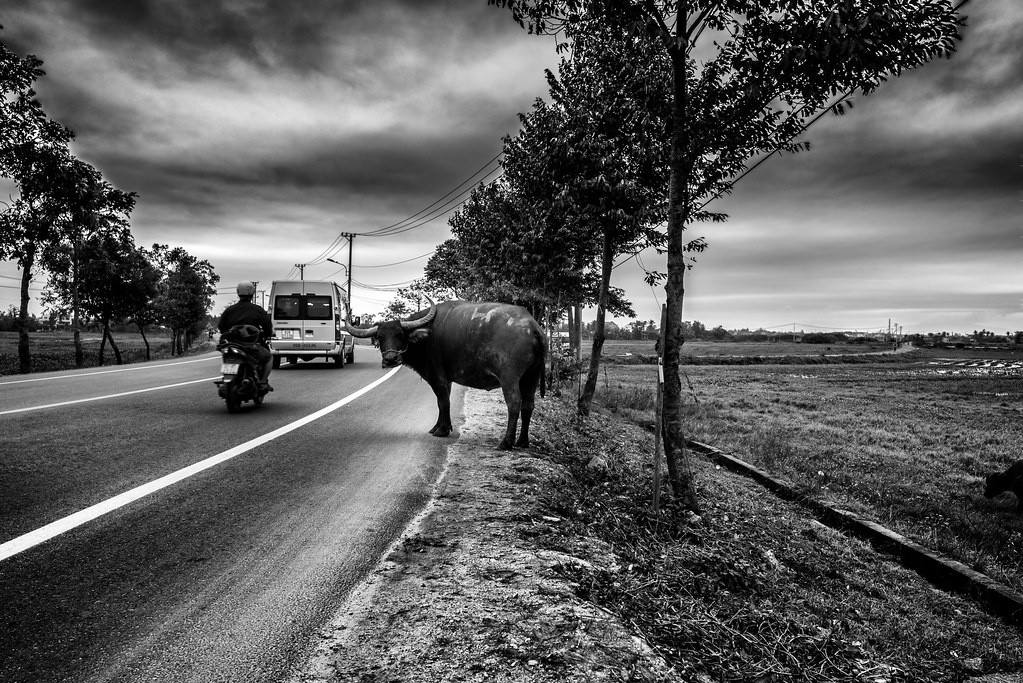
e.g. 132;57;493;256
344;294;550;450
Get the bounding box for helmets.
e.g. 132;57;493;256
236;281;255;295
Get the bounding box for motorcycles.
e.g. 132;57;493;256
213;305;272;414
209;329;213;338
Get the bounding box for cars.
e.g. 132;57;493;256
208;327;216;334
215;328;219;332
205;330;208;332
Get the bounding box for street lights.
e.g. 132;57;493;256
327;232;356;323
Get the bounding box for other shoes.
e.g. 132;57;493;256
260;380;273;392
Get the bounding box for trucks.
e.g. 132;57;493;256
268;281;354;368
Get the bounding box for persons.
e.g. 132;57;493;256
218;281;274;391
